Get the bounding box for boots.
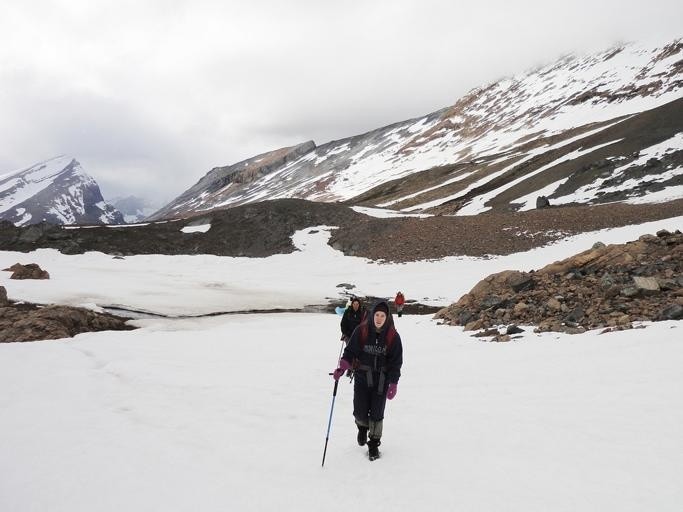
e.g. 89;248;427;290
355;420;369;446
367;434;381;461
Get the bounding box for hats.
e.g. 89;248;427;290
373;302;388;318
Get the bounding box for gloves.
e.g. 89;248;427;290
333;359;352;380
387;383;397;399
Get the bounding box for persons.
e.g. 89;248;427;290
334;301;402;459
341;299;364;378
345;297;353;308
394;292;405;317
